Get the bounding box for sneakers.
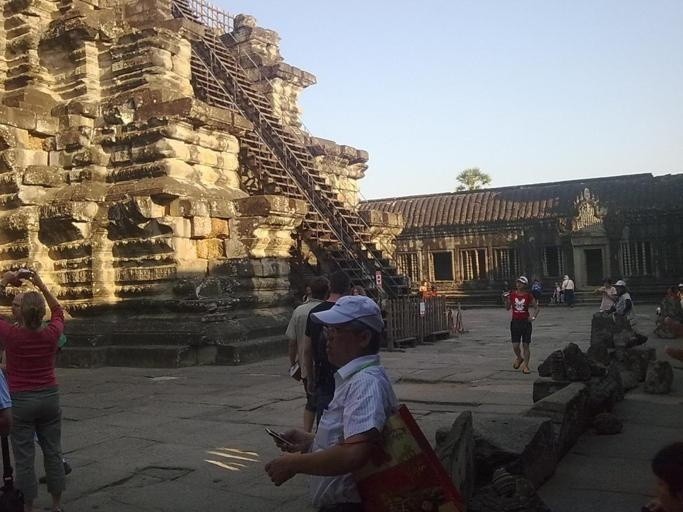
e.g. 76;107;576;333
38;463;71;483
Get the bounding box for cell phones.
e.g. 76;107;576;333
265;427;293;447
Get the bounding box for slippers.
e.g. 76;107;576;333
522;365;530;374
513;356;524;369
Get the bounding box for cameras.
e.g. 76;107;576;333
20;269;32;277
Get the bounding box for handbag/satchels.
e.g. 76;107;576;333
338;403;463;512
0;433;24;512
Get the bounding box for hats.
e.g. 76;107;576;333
517;276;528;285
611;280;627;288
310;295;385;335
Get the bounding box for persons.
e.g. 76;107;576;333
640;440;683;512
0;268;65;512
263;271;398;511
2;292;72;483
0;367;14;434
502;274;683;374
418;281;428;292
